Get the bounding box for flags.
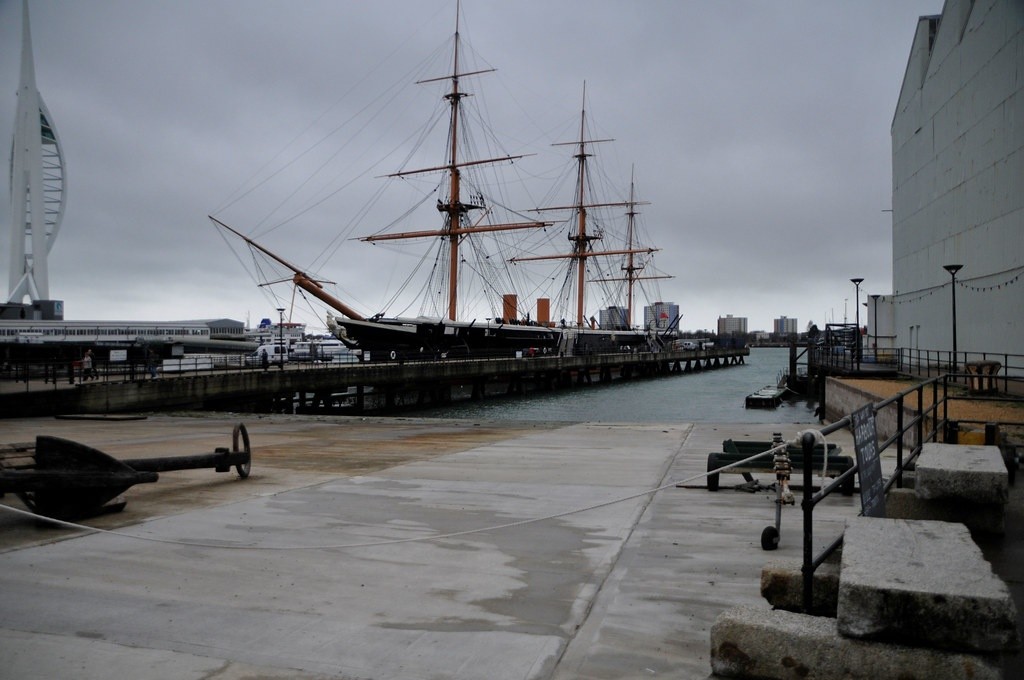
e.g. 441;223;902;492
659;312;670;318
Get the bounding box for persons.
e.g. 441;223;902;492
434;347;449;361
261;349;269;371
81;350;99;381
149;351;159;379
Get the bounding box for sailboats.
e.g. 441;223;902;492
208;0;683;362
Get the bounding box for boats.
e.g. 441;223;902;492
288;343;333;362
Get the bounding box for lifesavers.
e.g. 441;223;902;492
391;351;396;360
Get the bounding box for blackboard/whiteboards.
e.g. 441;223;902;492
851;402;887;516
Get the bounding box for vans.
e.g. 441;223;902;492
246;344;288;364
684;342;696;350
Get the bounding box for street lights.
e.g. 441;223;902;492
849;279;865;370
941;265;963;381
